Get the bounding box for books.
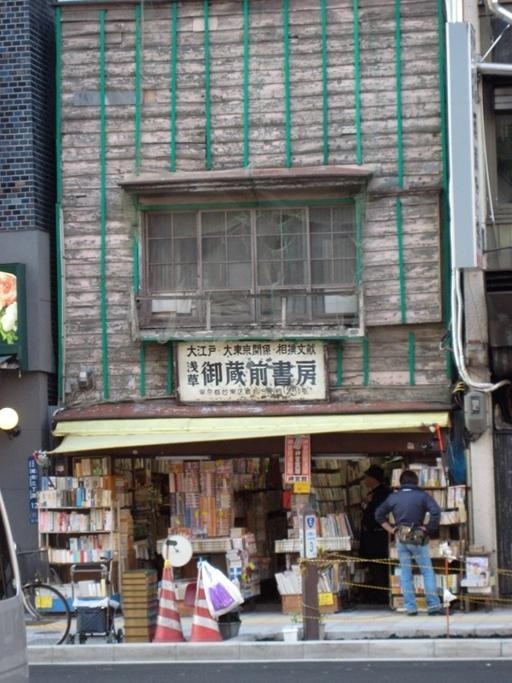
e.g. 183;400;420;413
34;455;287;595
280;456;490;595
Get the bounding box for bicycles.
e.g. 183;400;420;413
0;547;70;646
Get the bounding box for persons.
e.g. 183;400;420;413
374;470;453;616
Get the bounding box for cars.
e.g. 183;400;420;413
1;491;29;683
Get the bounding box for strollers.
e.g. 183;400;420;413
65;563;123;644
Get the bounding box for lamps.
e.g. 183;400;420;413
0;408;21;441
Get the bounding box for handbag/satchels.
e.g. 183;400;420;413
397;523;428;545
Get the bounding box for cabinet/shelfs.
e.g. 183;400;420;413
35;453;493;616
